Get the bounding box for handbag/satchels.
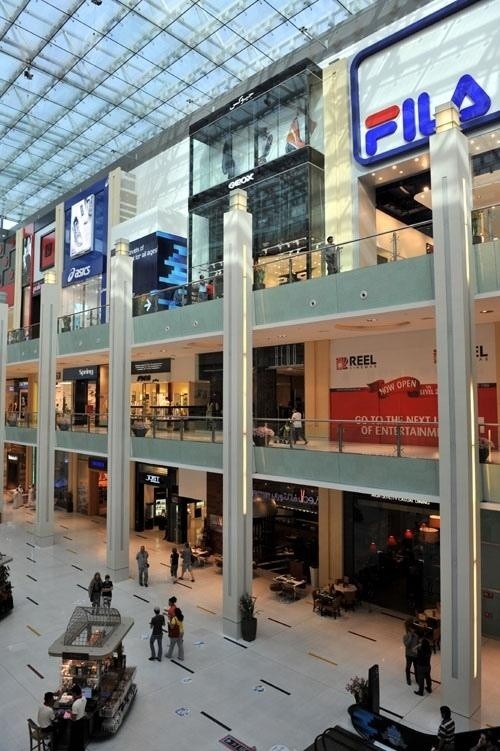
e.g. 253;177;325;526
168;624;180;638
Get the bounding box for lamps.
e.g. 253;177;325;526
432;101;460;137
43;267;58;285
113;236;131;259
227;187;246;214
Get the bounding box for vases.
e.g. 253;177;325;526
61;423;70;431
479;446;490;466
252;433;271;447
135;427;150;437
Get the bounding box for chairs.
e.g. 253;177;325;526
171;535;444;660
24;714;57;750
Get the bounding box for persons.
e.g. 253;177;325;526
199;275;207;301
38;541;195;751
206;399;214;430
470;730;500;751
55;404;62;413
166;411;174;439
13;484;36;510
64;404;70;413
207;280;216;300
288;408;309;445
325;236;336;275
431;706;455;751
413;639;432;696
404;627;419;685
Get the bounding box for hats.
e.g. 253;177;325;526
153;606;161;611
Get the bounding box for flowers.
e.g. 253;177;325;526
128;419;150;427
253;425;273;436
477;437;496;448
58;413;75;423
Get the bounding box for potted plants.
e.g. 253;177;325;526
345;675;370;716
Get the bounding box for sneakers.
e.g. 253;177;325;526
425;686;432;694
178;576;184;580
190;579;195;582
406;679;412;686
148;656;156;660
156;656;162;662
164;654;172;659
176;656;184;661
139;581;144;587
413;689;424;696
144;583;149;587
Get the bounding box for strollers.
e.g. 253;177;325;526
273;416;293;443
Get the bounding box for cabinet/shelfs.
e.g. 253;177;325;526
127;379;214;430
